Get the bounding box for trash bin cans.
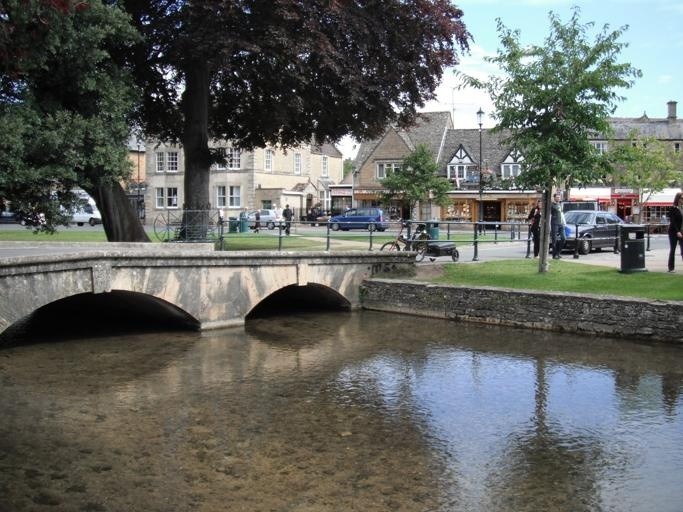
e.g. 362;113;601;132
240;211;248;232
620;225;648;273
229;217;237;232
427;221;439;240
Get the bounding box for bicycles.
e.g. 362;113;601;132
379;221;428;263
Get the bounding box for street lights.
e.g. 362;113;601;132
476;106;485;234
135;141;142;217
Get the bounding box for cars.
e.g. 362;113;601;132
0;201;27;226
236;209;286;230
549;209;625;255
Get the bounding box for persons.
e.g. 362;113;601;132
549;194;566;259
282;204;292;236
242;207;248;218
528;200;542;258
253;210;261;233
667;192;682;273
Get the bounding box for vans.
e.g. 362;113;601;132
50;190;102;226
327;207;389;232
